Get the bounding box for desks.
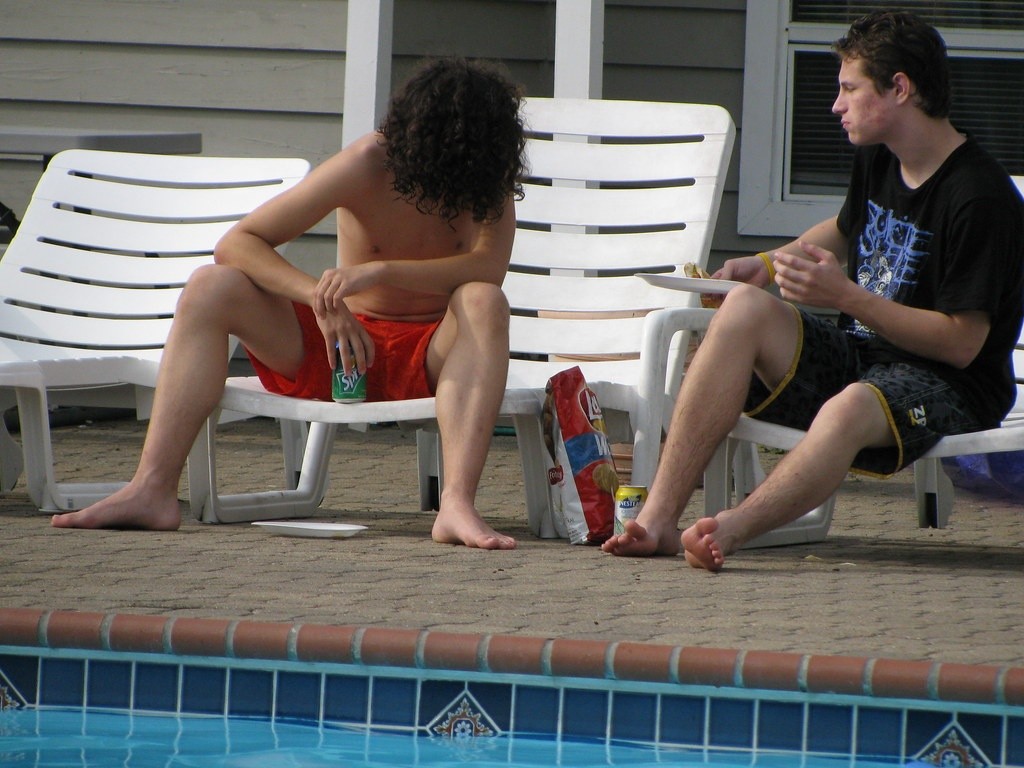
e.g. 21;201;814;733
0;123;204;428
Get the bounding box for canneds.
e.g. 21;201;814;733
614;485;649;535
331;339;368;403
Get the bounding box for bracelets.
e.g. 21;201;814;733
756;253;775;288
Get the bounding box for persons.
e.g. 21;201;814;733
602;10;1024;570
51;56;531;547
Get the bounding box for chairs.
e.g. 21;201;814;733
0;148;310;512
187;96;737;540
627;173;1024;553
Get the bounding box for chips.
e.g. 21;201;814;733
592;463;620;493
